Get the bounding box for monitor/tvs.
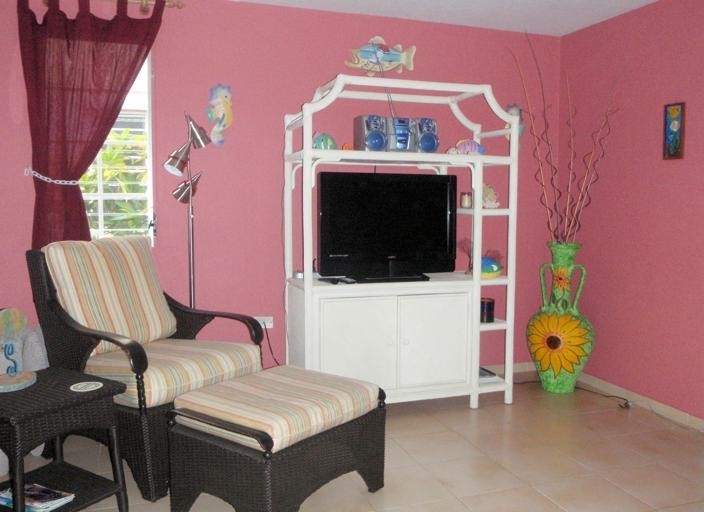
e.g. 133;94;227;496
315;172;459;283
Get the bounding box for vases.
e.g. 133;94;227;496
526;239;597;396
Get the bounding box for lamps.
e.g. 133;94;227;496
162;110;212;312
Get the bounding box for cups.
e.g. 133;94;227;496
480;297;495;324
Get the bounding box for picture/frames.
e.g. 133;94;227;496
662;101;684;160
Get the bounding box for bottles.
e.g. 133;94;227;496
460;191;472;209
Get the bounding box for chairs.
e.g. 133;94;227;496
285;71;522;412
24;234;265;504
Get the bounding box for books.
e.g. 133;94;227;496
0;482;75;512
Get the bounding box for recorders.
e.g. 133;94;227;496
353;114;439;153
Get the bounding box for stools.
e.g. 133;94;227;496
167;364;387;511
